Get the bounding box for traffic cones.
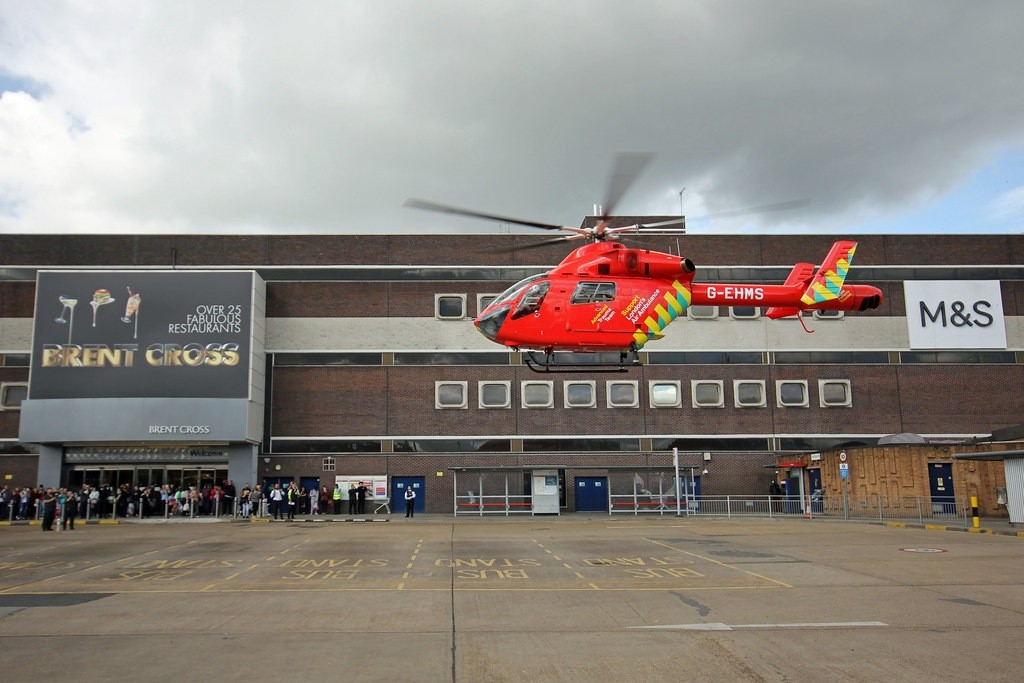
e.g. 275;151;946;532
804;501;810;519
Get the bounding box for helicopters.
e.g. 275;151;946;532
403;150;884;373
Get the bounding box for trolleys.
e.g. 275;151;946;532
373;498;391;514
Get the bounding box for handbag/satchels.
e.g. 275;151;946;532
183;503;190;511
313;501;319;510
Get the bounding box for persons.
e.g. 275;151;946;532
525;284;543;310
0;478;368;532
404;485;417;517
